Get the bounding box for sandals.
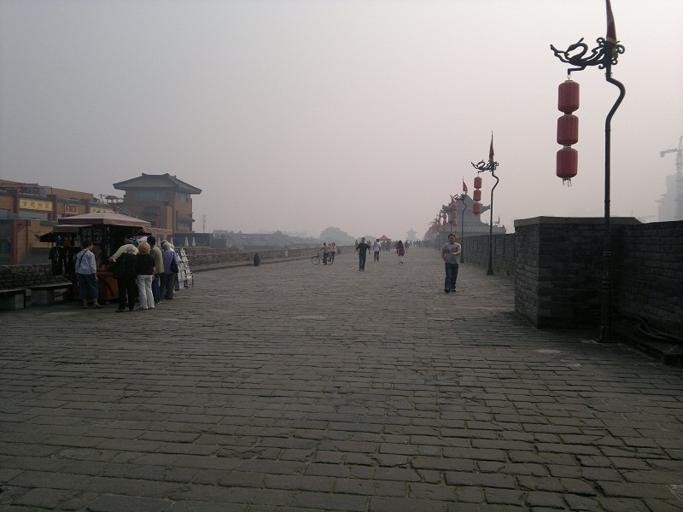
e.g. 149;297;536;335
82;304;104;309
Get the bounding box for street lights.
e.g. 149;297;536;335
550;2;624;216
471;131;498;276
424;177;468;263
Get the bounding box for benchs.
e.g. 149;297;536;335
28;283;72;304
0;288;31;309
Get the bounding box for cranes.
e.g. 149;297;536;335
660;136;683;181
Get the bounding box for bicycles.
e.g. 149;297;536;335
312;249;333;265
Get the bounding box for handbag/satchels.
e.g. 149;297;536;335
170;252;179;273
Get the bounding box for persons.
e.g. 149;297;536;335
355;236;370;273
352;234;424;254
439;233;461;294
395;239;405;264
73;238;106;310
330;242;336;264
371;238;381;265
319;242;330;265
107;234;178;313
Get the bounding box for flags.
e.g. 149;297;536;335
461;178;467;196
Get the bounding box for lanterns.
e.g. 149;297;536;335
556;114;579;147
556;77;580;114
473;189;481;202
472;202;480;216
473;175;482;188
422;198;457;243
555;148;578;188
